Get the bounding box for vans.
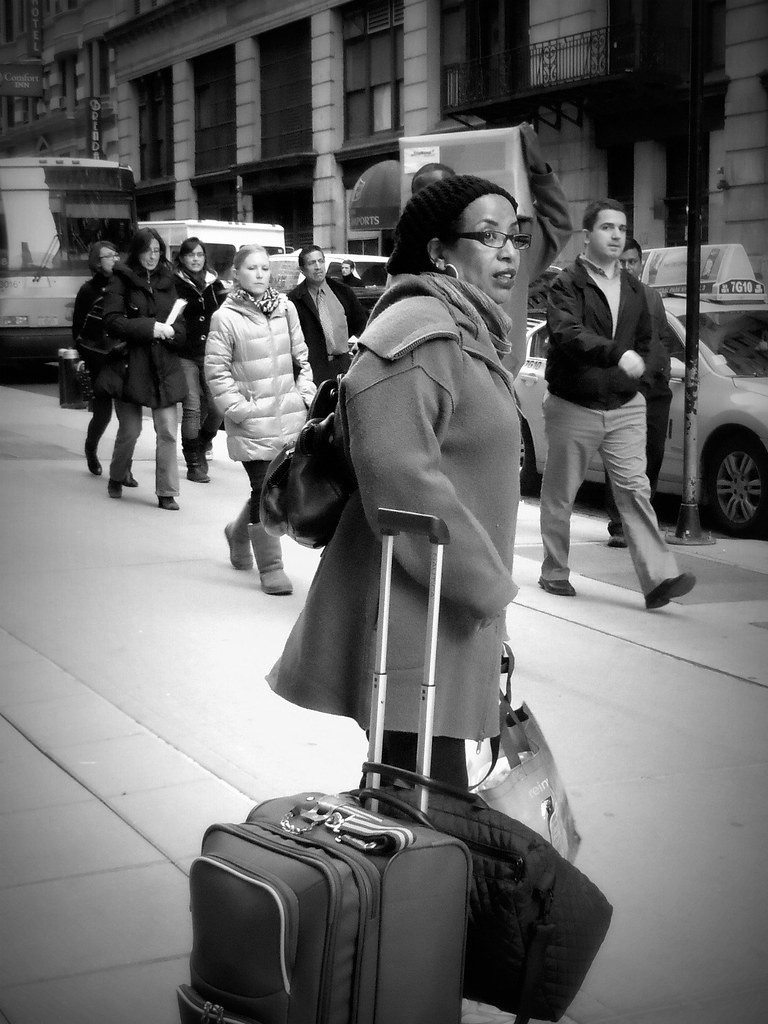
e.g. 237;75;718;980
137;218;284;282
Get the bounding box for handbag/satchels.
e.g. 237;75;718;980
345;761;614;1020
479;645;582;863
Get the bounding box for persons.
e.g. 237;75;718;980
338;259;363;288
540;200;696;609
605;238;672;547
412;163;456;198
287;246;369;387
104;228;187;512
268;177;522;799
205;241;316;594
174;235;228;485
72;240;140;488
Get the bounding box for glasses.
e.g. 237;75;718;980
99;252;120;261
143;248;159;256
459;231;531;250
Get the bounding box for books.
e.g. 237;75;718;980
165;298;188;325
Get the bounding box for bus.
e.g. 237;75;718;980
0;157;138;369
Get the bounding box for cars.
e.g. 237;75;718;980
262;249;394;322
510;242;768;542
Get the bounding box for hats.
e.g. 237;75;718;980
385;176;518;276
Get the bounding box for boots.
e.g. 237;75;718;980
225;498;253;569
247;523;292;594
182;448;212;483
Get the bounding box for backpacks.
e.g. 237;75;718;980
259;375;356;549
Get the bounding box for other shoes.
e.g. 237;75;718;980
123;473;138;488
108;477;122;498
85;448;102;475
206;451;213;460
609;535;627;548
158;496;180;510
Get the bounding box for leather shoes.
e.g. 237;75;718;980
538;576;576;596
644;574;696;609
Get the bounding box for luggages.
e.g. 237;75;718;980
178;508;474;1024
